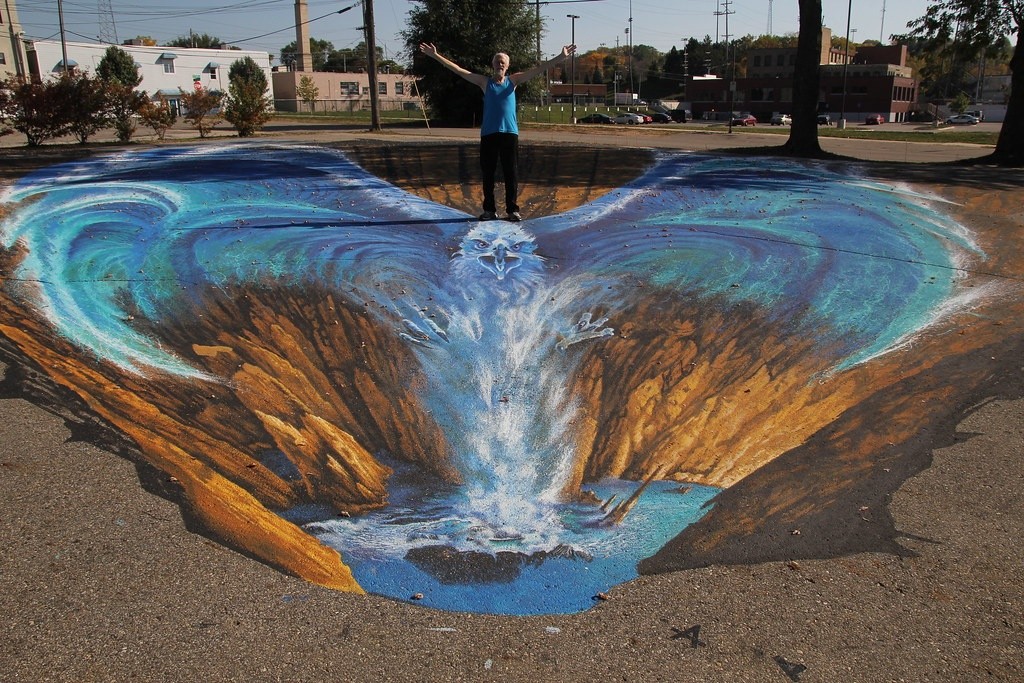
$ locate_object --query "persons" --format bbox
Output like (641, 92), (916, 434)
(420, 42), (578, 221)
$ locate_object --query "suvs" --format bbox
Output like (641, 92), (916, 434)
(959, 110), (985, 122)
(665, 109), (692, 123)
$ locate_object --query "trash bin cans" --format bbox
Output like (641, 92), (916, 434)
(974, 111), (983, 121)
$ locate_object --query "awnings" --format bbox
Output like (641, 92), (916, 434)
(208, 62), (218, 68)
(59, 60), (78, 66)
(159, 88), (181, 95)
(161, 53), (177, 59)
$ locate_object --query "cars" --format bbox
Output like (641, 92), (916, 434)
(577, 113), (615, 124)
(943, 114), (979, 124)
(817, 115), (834, 126)
(613, 113), (672, 124)
(770, 114), (792, 125)
(865, 114), (884, 125)
(732, 114), (759, 126)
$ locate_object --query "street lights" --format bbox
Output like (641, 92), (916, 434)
(567, 14), (580, 124)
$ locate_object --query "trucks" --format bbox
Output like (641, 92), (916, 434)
(615, 93), (647, 105)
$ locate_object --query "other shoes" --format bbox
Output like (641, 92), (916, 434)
(507, 212), (522, 221)
(479, 210), (497, 220)
